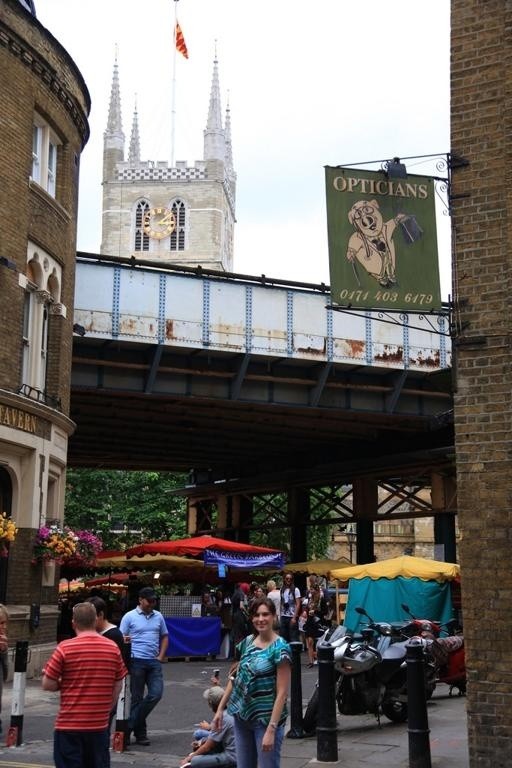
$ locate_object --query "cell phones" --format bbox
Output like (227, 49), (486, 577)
(213, 670), (220, 678)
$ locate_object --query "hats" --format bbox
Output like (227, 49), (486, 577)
(202, 685), (226, 704)
(137, 585), (160, 601)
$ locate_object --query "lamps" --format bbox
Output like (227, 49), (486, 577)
(73, 319), (86, 336)
(1, 256), (17, 270)
(383, 156), (411, 181)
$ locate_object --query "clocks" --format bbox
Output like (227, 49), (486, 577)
(141, 205), (177, 241)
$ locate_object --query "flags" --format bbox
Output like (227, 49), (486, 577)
(173, 20), (189, 60)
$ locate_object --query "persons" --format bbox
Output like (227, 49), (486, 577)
(120, 586), (170, 745)
(212, 594), (294, 768)
(202, 573), (333, 669)
(179, 686), (236, 768)
(346, 198), (406, 288)
(85, 596), (124, 747)
(0, 603), (10, 734)
(192, 661), (238, 740)
(41, 601), (123, 768)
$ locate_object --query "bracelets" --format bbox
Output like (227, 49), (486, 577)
(269, 721), (278, 729)
(188, 752), (194, 757)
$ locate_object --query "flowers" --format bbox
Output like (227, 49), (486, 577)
(71, 529), (104, 572)
(1, 513), (20, 558)
(29, 522), (80, 567)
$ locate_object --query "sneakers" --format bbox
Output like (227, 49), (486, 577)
(123, 726), (133, 747)
(135, 736), (152, 746)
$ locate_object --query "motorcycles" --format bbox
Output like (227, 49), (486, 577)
(302, 604), (466, 735)
